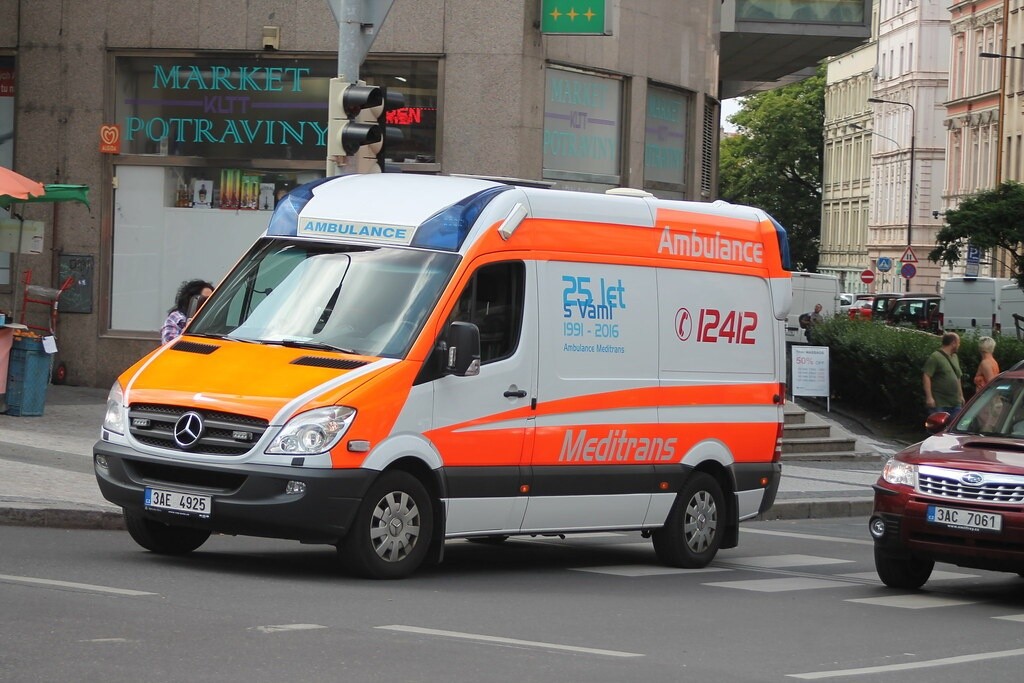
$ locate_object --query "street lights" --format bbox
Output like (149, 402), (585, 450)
(326, 78), (383, 180)
(867, 97), (915, 293)
(845, 123), (906, 249)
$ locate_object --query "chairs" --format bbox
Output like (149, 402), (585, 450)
(914, 307), (921, 317)
(900, 306), (906, 315)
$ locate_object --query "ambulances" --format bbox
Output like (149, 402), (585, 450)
(93, 163), (791, 579)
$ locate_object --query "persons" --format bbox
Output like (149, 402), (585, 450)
(921, 332), (966, 426)
(155, 276), (216, 347)
(799, 304), (823, 344)
(974, 336), (1001, 394)
(992, 321), (1003, 334)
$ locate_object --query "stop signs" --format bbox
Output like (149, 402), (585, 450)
(861, 270), (875, 283)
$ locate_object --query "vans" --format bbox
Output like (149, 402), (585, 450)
(938, 277), (1024, 339)
(786, 271), (840, 344)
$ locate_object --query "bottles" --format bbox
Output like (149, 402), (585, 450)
(176, 184), (188, 207)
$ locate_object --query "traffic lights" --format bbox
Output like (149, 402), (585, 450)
(380, 85), (404, 174)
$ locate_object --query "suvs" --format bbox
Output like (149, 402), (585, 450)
(839, 291), (941, 334)
(868, 360), (1024, 592)
